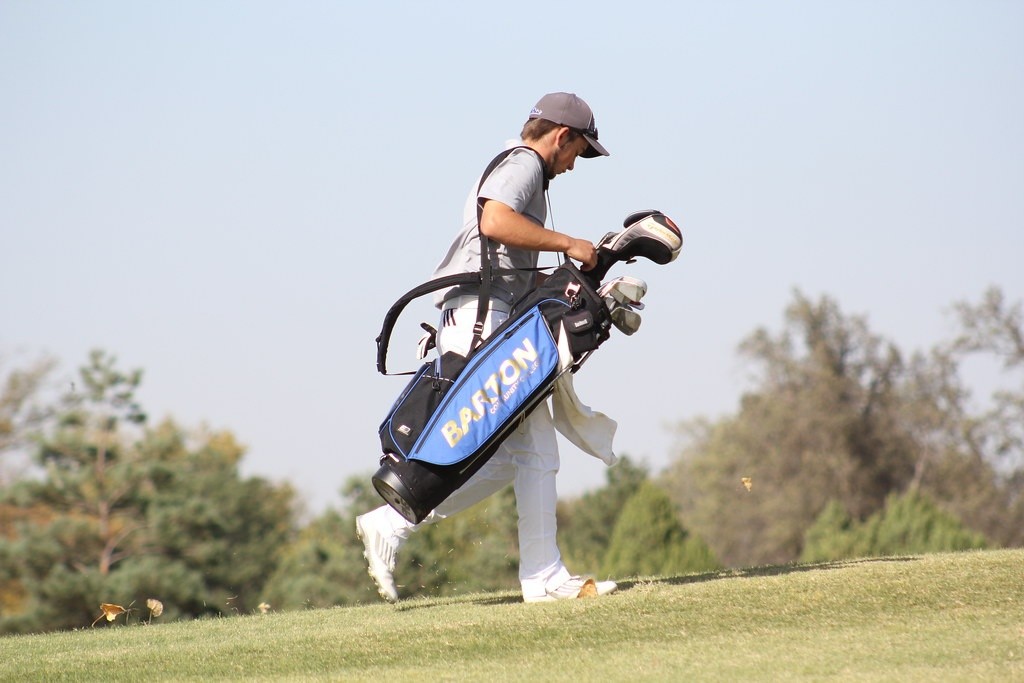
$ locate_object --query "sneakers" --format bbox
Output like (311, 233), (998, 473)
(524, 576), (617, 603)
(355, 515), (398, 604)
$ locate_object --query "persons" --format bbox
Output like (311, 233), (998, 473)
(356, 92), (622, 604)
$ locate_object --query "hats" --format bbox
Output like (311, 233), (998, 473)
(528, 91), (611, 156)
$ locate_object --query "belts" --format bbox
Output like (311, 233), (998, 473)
(441, 296), (516, 315)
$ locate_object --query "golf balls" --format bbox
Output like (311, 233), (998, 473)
(595, 275), (648, 335)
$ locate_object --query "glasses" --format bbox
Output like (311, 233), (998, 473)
(561, 124), (598, 139)
